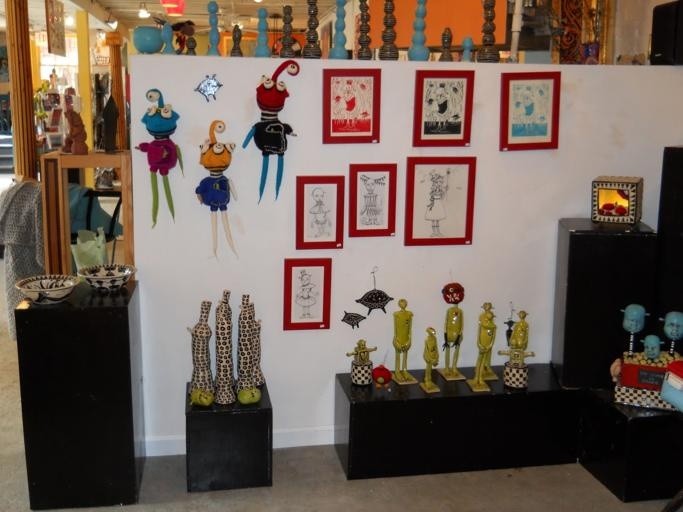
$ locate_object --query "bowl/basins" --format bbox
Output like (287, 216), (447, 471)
(77, 265), (137, 291)
(15, 274), (79, 304)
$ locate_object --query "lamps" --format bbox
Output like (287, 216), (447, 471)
(160, 0), (184, 16)
(138, 3), (151, 18)
(105, 13), (118, 29)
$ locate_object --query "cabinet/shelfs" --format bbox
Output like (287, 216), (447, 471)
(405, 155), (476, 245)
(296, 175), (345, 250)
(500, 71), (562, 151)
(413, 70), (475, 147)
(336, 360), (580, 479)
(323, 68), (380, 144)
(284, 258), (332, 331)
(349, 164), (396, 237)
(548, 221), (682, 502)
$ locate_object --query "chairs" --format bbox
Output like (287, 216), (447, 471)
(71, 191), (122, 265)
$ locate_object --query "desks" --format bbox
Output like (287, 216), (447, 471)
(186, 379), (273, 493)
(13, 281), (146, 510)
